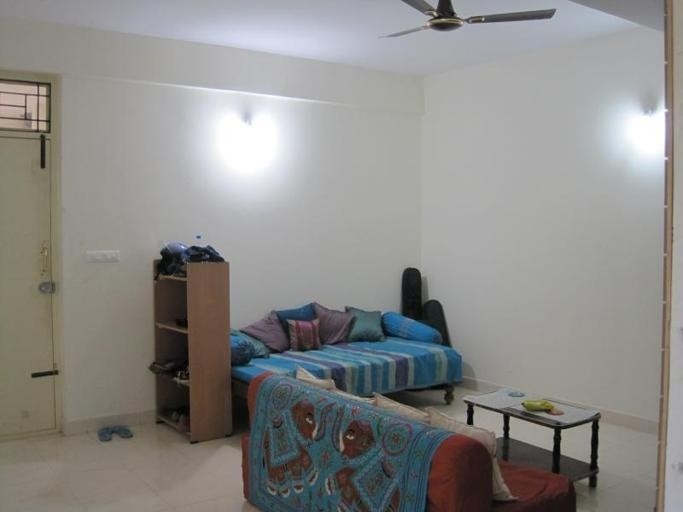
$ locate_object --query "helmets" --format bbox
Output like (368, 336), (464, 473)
(160, 242), (188, 260)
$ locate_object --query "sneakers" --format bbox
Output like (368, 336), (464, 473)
(170, 407), (189, 424)
(172, 368), (189, 384)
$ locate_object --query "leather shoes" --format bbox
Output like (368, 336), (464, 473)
(148, 358), (189, 376)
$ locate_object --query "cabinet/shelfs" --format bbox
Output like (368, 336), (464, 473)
(153, 257), (232, 445)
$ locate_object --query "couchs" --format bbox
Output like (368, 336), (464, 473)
(242, 374), (576, 512)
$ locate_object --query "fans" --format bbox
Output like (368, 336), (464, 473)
(378, 0), (556, 40)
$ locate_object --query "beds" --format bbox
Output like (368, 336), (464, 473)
(232, 335), (462, 405)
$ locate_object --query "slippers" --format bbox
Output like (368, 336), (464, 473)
(112, 425), (133, 438)
(97, 426), (112, 442)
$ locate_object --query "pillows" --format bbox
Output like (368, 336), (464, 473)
(295, 363), (519, 502)
(230, 267), (444, 364)
